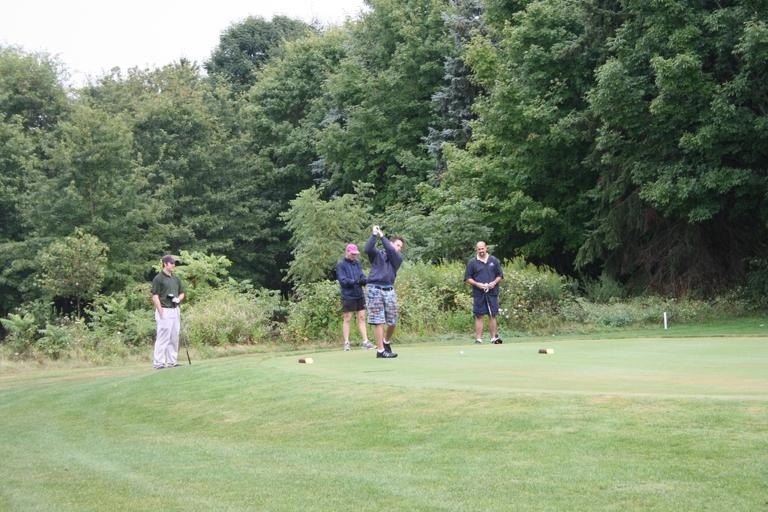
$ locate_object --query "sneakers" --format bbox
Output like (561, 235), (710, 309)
(377, 350), (398, 358)
(154, 363), (183, 369)
(383, 338), (392, 353)
(476, 338), (498, 344)
(361, 341), (377, 349)
(344, 342), (350, 351)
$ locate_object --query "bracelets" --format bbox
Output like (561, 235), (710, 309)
(493, 281), (497, 286)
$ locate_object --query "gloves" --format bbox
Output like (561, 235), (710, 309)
(359, 279), (367, 286)
(172, 297), (180, 304)
(373, 226), (380, 236)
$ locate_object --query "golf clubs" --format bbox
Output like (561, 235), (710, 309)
(483, 289), (504, 345)
(167, 292), (192, 365)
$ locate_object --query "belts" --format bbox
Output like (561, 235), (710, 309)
(367, 284), (393, 291)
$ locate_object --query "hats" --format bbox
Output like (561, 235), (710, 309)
(345, 244), (360, 255)
(162, 255), (177, 264)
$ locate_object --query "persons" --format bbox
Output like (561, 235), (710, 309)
(333, 242), (377, 352)
(461, 239), (505, 345)
(362, 223), (406, 360)
(148, 253), (189, 369)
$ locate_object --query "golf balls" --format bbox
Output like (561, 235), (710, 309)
(459, 350), (465, 355)
